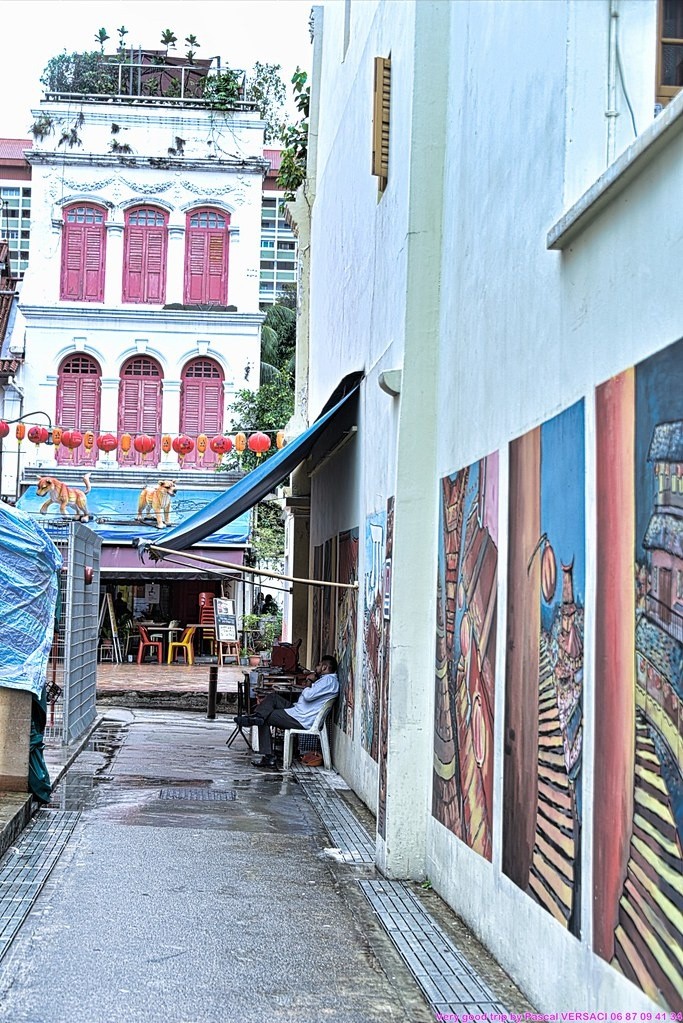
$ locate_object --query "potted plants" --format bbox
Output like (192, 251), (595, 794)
(238, 84), (244, 94)
(275, 624), (282, 641)
(239, 647), (249, 665)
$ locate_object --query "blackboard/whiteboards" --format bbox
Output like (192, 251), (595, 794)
(213, 598), (239, 642)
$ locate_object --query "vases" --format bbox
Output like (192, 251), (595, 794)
(249, 655), (261, 667)
(262, 659), (271, 667)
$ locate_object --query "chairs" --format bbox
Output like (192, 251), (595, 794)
(199, 592), (215, 655)
(136, 620), (162, 665)
(225, 670), (335, 772)
(167, 626), (196, 665)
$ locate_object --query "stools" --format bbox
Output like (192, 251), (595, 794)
(100, 644), (124, 662)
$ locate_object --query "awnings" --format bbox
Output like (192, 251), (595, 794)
(135, 385), (361, 564)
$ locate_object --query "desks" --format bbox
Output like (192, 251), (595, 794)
(134, 622), (185, 664)
(238, 630), (261, 653)
(253, 682), (306, 702)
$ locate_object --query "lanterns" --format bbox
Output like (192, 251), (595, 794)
(1, 417), (285, 459)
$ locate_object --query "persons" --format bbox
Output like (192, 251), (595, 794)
(113, 591), (132, 619)
(262, 596), (278, 616)
(252, 592), (265, 615)
(234, 654), (341, 768)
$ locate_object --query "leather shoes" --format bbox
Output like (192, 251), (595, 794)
(251, 756), (276, 769)
(233, 715), (264, 728)
